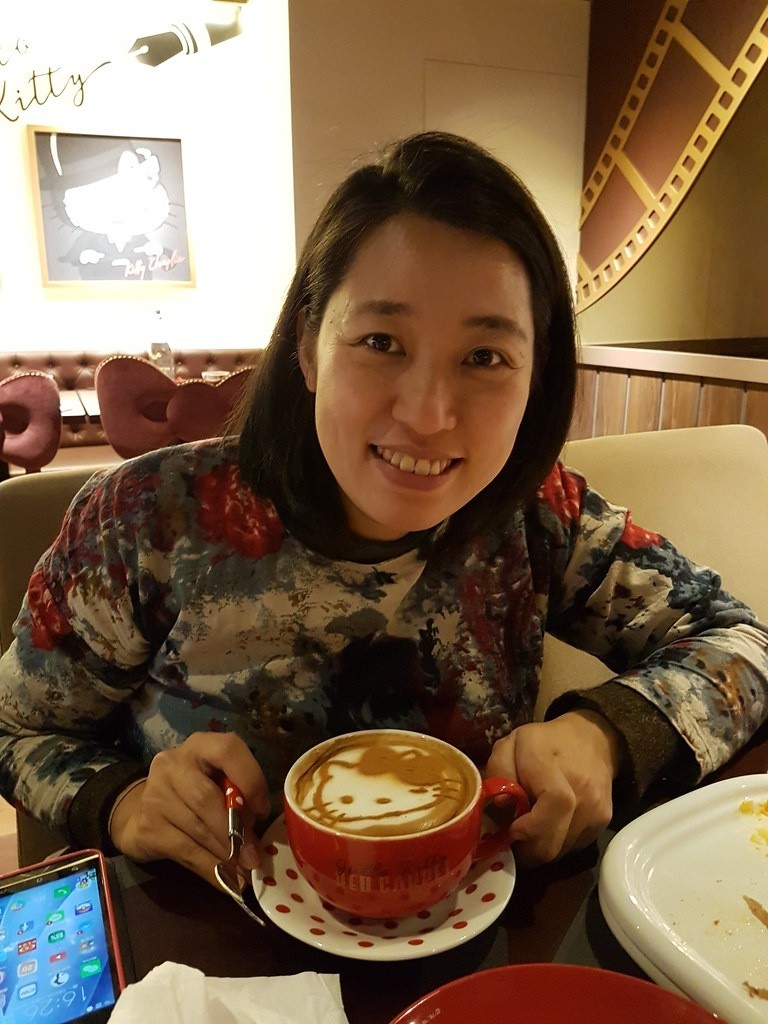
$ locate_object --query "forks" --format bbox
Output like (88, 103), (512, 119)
(213, 774), (266, 927)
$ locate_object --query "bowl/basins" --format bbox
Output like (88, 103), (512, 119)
(386, 964), (725, 1024)
(201, 371), (229, 382)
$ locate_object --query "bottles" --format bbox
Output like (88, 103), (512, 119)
(147, 309), (175, 383)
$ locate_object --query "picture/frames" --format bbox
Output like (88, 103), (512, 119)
(23, 123), (198, 292)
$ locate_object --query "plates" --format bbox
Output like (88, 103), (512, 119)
(250, 813), (517, 962)
(596, 771), (768, 1024)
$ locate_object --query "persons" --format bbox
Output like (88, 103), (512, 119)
(0, 129), (768, 895)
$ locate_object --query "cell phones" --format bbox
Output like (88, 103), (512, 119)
(0, 851), (137, 1024)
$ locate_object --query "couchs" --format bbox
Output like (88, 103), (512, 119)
(0, 349), (264, 449)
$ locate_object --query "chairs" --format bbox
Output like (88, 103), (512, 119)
(0, 467), (109, 661)
(0, 372), (62, 482)
(95, 356), (255, 461)
(530, 424), (768, 726)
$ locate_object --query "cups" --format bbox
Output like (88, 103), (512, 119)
(282, 728), (530, 920)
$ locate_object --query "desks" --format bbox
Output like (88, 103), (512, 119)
(56, 725), (768, 1024)
